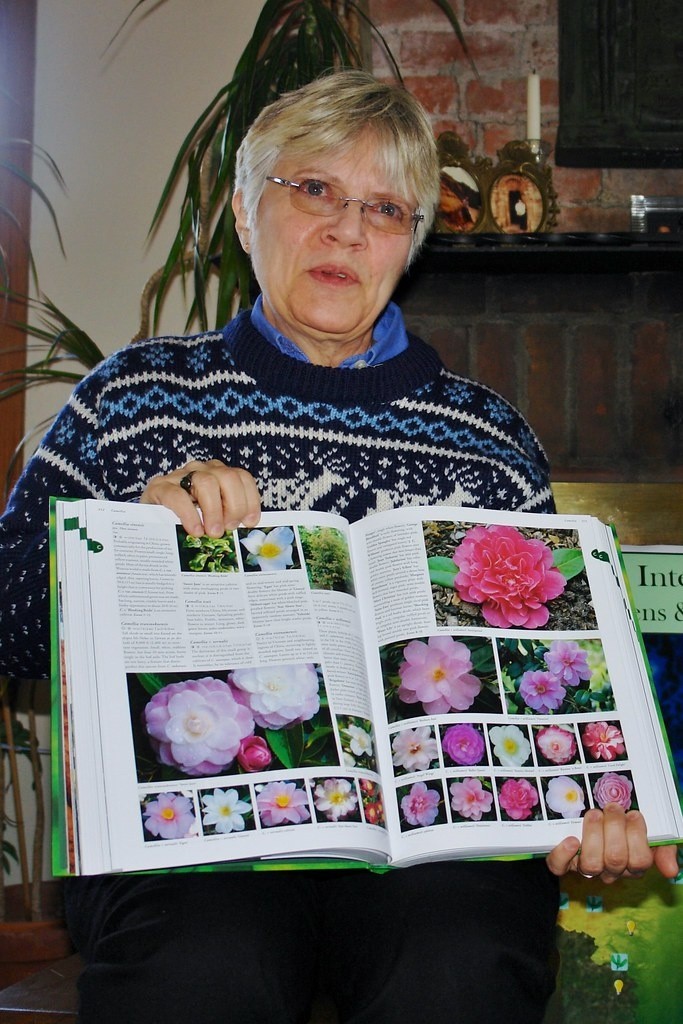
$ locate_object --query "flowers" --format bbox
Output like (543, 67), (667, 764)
(427, 525), (585, 629)
(138, 663), (386, 840)
(237, 526), (302, 572)
(381, 636), (640, 826)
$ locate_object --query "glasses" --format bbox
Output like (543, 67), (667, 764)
(264, 175), (424, 240)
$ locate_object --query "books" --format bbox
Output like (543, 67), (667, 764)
(49, 497), (683, 878)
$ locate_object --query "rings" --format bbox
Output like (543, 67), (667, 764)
(180, 471), (198, 495)
(577, 863), (601, 879)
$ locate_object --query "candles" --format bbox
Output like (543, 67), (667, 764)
(527, 68), (541, 163)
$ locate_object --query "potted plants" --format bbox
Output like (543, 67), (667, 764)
(0, 0), (480, 1024)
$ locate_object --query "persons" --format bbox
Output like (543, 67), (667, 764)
(514, 198), (526, 229)
(439, 178), (475, 231)
(0, 70), (680, 1024)
